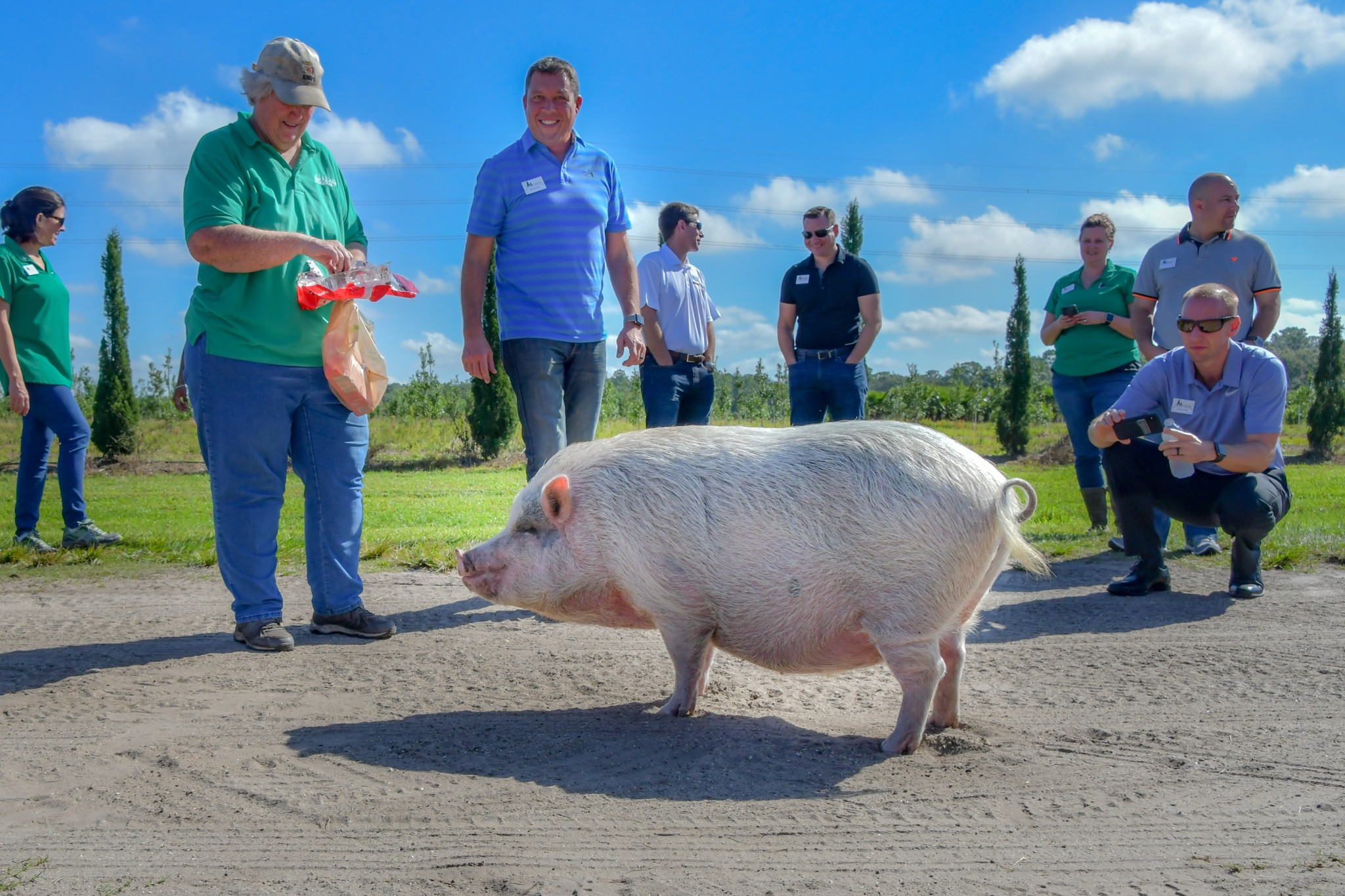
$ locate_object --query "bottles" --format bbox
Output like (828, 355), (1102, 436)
(1161, 419), (1194, 479)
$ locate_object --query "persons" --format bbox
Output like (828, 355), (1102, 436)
(173, 37), (396, 652)
(460, 58), (646, 480)
(638, 201), (721, 427)
(1040, 212), (1137, 533)
(1, 185), (124, 550)
(777, 207), (882, 426)
(1130, 172), (1282, 363)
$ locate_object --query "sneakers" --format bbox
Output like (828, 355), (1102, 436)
(13, 530), (58, 553)
(1106, 536), (1124, 549)
(310, 605), (398, 639)
(61, 519), (124, 549)
(234, 619), (295, 652)
(1186, 535), (1222, 556)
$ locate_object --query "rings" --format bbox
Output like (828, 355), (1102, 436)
(1084, 319), (1087, 323)
(640, 356), (643, 358)
(1177, 447), (1180, 455)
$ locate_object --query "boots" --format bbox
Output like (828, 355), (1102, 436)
(1079, 488), (1109, 536)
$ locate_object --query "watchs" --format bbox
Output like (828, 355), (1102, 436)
(1208, 442), (1226, 463)
(703, 362), (715, 369)
(623, 313), (645, 327)
(1088, 283), (1291, 601)
(1104, 312), (1114, 326)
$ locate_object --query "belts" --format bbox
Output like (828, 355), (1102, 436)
(797, 343), (853, 359)
(669, 350), (706, 363)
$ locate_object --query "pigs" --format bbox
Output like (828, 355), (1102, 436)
(461, 415), (1050, 755)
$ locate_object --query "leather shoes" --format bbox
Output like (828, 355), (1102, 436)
(1108, 561), (1172, 596)
(1228, 558), (1265, 597)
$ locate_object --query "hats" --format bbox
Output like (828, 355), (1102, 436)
(253, 37), (332, 111)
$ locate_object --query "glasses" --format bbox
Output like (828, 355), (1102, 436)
(687, 220), (702, 230)
(1176, 314), (1237, 333)
(802, 226), (834, 238)
(48, 215), (66, 225)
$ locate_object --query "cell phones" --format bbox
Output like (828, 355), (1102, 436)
(1111, 414), (1163, 440)
(1061, 304), (1079, 316)
